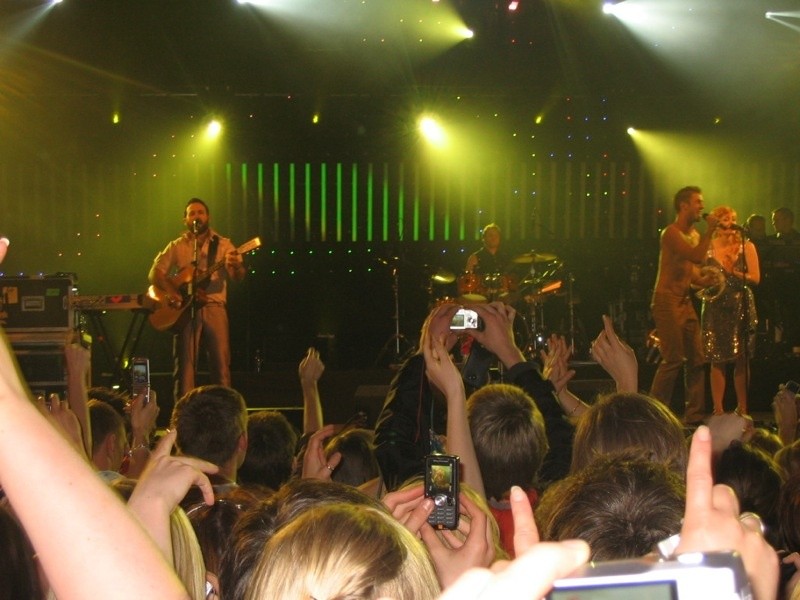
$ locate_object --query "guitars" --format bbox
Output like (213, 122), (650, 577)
(144, 237), (262, 333)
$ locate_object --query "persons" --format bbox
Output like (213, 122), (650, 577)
(650, 185), (720, 426)
(690, 205), (760, 418)
(149, 198), (244, 403)
(466, 223), (513, 275)
(0, 237), (800, 600)
(746, 208), (800, 354)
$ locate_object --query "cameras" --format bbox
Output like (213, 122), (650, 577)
(446, 309), (479, 330)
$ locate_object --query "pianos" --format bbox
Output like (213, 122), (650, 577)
(71, 290), (156, 389)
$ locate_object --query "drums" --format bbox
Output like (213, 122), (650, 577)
(459, 272), (491, 303)
(487, 271), (513, 297)
(513, 309), (532, 356)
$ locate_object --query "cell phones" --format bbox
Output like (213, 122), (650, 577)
(424, 453), (460, 531)
(132, 358), (150, 403)
(543, 548), (757, 600)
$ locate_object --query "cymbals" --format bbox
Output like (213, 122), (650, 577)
(375, 254), (416, 273)
(431, 263), (459, 285)
(694, 264), (726, 303)
(523, 278), (562, 304)
(511, 249), (557, 267)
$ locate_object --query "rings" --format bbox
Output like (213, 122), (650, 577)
(740, 513), (765, 534)
(325, 463), (334, 472)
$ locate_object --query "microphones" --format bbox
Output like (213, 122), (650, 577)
(192, 219), (200, 233)
(729, 224), (760, 240)
(702, 213), (726, 229)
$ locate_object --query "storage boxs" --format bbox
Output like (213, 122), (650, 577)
(0, 272), (78, 344)
